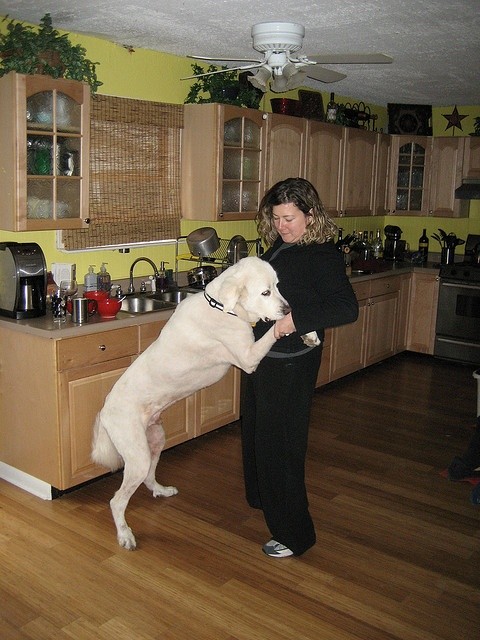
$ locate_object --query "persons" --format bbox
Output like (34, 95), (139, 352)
(239, 178), (358, 557)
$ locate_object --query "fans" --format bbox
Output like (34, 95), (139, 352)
(175, 21), (394, 91)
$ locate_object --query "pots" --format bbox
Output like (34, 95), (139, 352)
(185, 227), (221, 258)
(187, 265), (218, 289)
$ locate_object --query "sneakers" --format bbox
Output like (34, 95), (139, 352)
(262, 540), (294, 558)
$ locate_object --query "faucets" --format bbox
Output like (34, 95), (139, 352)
(128, 257), (159, 292)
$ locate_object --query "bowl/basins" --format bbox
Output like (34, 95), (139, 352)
(67, 299), (98, 317)
(95, 299), (122, 319)
(83, 291), (111, 311)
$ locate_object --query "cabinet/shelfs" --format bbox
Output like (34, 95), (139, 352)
(404, 265), (439, 356)
(264, 115), (344, 222)
(464, 133), (480, 183)
(1, 72), (93, 233)
(345, 124), (389, 217)
(4, 308), (240, 490)
(390, 134), (432, 217)
(431, 136), (470, 218)
(316, 267), (409, 388)
(180, 102), (262, 223)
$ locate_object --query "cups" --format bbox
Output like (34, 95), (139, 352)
(441, 247), (455, 266)
(50, 288), (68, 323)
(71, 297), (98, 324)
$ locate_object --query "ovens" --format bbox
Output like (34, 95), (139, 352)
(433, 283), (480, 367)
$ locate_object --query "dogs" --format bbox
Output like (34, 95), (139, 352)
(88, 255), (301, 552)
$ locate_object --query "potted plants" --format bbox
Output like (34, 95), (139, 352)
(1, 13), (104, 96)
(183, 61), (264, 111)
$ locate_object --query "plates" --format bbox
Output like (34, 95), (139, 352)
(226, 235), (249, 263)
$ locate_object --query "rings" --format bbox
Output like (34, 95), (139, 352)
(285, 334), (291, 337)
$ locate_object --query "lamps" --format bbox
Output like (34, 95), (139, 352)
(245, 49), (313, 94)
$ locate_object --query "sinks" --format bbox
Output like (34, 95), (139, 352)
(120, 295), (176, 317)
(153, 289), (199, 304)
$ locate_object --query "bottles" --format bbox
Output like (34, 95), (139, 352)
(368, 230), (376, 258)
(418, 228), (429, 257)
(357, 231), (365, 262)
(363, 230), (372, 260)
(325, 92), (338, 123)
(335, 227), (347, 255)
(374, 228), (383, 254)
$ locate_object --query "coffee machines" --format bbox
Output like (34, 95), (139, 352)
(0, 241), (48, 320)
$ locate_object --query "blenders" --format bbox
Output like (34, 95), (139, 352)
(383, 224), (407, 262)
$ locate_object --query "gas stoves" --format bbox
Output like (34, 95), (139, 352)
(438, 261), (479, 282)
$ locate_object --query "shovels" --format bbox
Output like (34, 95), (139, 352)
(449, 232), (457, 248)
(454, 238), (465, 248)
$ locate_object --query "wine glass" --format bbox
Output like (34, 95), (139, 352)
(60, 280), (78, 316)
